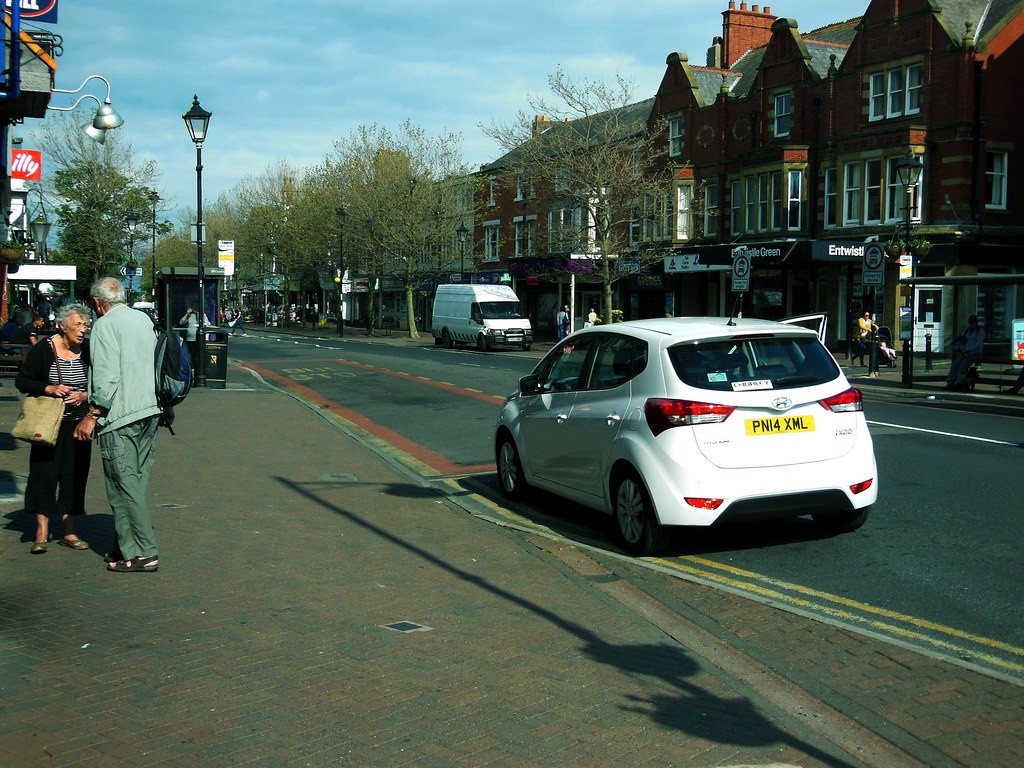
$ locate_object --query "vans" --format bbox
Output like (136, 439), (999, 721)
(431, 284), (535, 352)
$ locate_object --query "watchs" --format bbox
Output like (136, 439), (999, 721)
(87, 410), (100, 419)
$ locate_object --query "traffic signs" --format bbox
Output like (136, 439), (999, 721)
(120, 267), (143, 277)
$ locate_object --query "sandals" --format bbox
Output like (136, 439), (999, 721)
(107, 554), (159, 572)
(104, 551), (126, 563)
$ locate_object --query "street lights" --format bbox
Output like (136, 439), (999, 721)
(336, 208), (349, 333)
(895, 149), (924, 388)
(146, 189), (163, 302)
(181, 93), (212, 390)
(455, 222), (469, 283)
(126, 212), (140, 307)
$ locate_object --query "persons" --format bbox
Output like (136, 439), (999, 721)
(73, 278), (163, 572)
(0, 312), (44, 372)
(179, 302), (211, 379)
(661, 307), (672, 318)
(21, 295), (70, 330)
(945, 315), (985, 386)
(15, 303), (91, 553)
(220, 301), (308, 336)
(556, 305), (571, 342)
(850, 312), (896, 367)
(588, 308), (598, 323)
(1002, 367), (1024, 395)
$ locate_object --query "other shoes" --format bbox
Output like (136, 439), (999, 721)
(860, 365), (867, 368)
(31, 540), (48, 552)
(851, 358), (854, 364)
(59, 537), (89, 550)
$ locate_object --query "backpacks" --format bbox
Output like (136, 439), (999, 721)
(133, 308), (192, 406)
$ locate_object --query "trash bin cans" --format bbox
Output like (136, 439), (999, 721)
(194, 327), (228, 389)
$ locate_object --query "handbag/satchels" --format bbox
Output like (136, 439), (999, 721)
(10, 397), (64, 445)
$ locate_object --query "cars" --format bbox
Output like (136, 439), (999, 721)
(494, 316), (878, 557)
